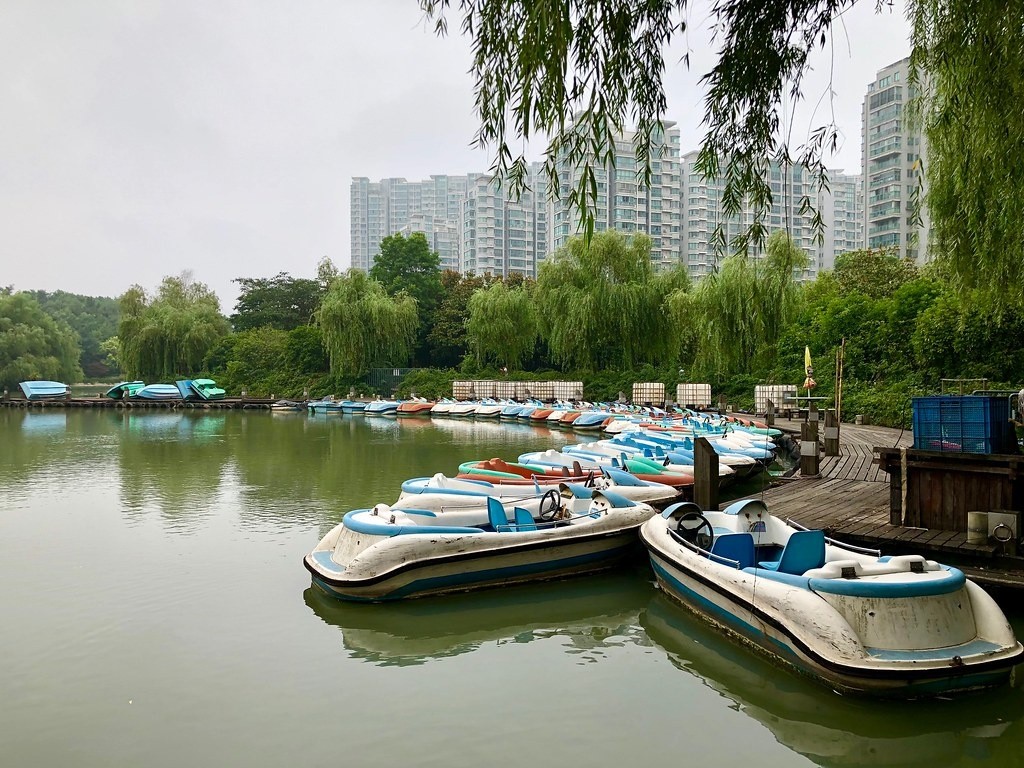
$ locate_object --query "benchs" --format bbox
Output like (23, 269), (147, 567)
(784, 406), (836, 423)
(801, 554), (941, 579)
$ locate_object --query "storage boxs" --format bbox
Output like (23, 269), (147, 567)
(911, 395), (1009, 455)
(988, 509), (1020, 540)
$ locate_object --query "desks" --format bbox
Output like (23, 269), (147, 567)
(787, 395), (832, 423)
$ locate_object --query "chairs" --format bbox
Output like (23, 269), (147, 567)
(368, 503), (419, 528)
(487, 497), (538, 533)
(708, 529), (826, 575)
(396, 398), (763, 495)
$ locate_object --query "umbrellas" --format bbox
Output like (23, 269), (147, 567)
(802, 345), (817, 420)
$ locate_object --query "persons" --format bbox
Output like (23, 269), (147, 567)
(500, 366), (508, 376)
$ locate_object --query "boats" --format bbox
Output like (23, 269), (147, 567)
(639, 591), (1013, 768)
(191, 377), (227, 400)
(136, 383), (183, 400)
(175, 380), (201, 401)
(301, 566), (653, 668)
(637, 497), (1024, 707)
(300, 506), (654, 602)
(18, 380), (71, 402)
(270, 401), (301, 411)
(306, 400), (785, 504)
(106, 381), (146, 400)
